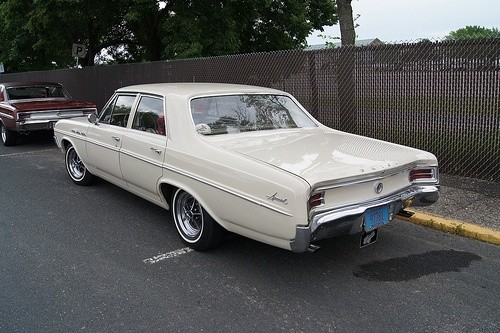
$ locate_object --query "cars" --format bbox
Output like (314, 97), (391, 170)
(54, 82), (440, 254)
(0, 81), (97, 148)
